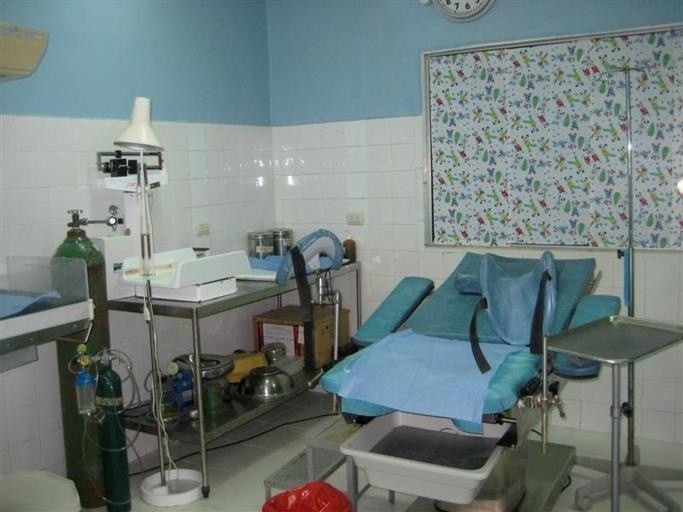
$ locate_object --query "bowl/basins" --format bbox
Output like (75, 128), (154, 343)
(235, 365), (294, 402)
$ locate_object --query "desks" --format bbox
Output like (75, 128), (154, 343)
(540, 312), (682, 511)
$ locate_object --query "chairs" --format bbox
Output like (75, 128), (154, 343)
(318, 250), (621, 511)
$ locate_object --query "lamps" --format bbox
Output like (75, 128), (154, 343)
(110, 95), (206, 510)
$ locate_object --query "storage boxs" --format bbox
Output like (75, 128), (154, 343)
(338, 411), (513, 505)
(251, 304), (350, 370)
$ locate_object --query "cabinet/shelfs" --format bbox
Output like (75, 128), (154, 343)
(106, 260), (363, 499)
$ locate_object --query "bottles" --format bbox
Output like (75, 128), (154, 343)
(152, 372), (184, 429)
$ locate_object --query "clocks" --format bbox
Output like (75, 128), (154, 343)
(418, 0), (495, 25)
